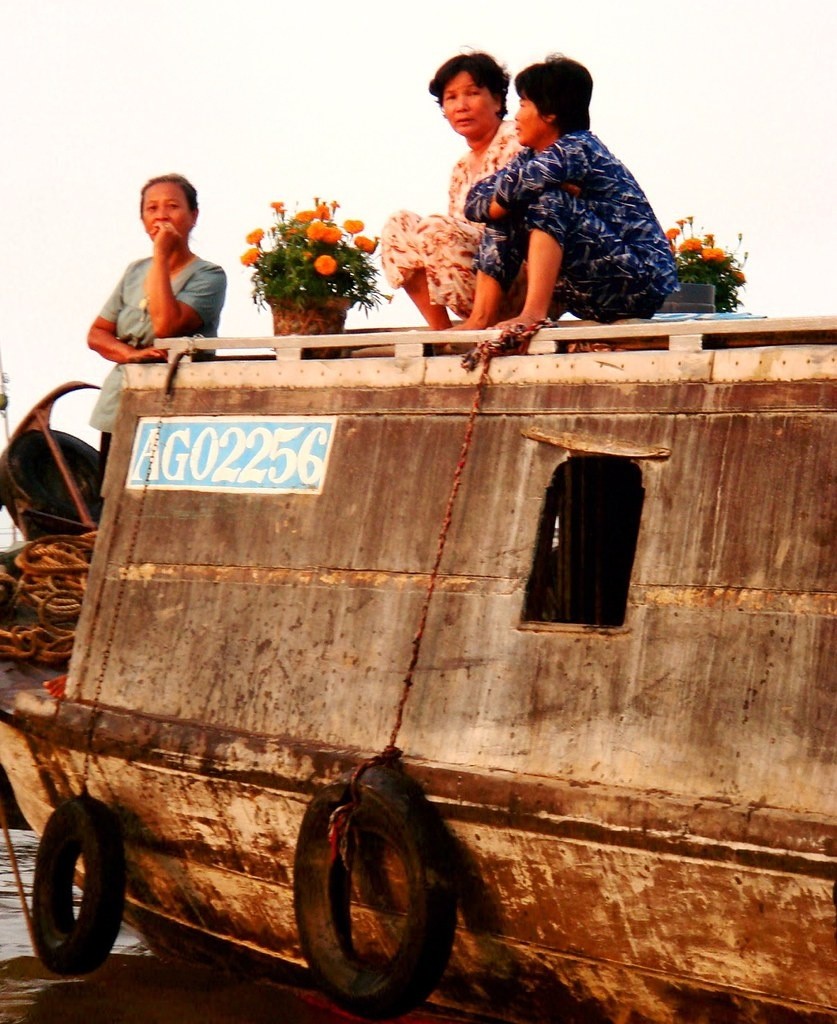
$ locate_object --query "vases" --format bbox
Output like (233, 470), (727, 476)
(652, 281), (716, 316)
(267, 296), (352, 361)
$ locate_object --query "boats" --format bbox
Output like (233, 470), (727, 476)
(0, 313), (837, 1024)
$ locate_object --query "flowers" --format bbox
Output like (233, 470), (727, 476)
(668, 215), (748, 314)
(240, 198), (394, 314)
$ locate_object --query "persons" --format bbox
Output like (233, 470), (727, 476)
(382, 51), (531, 331)
(438, 55), (677, 330)
(87, 174), (227, 503)
